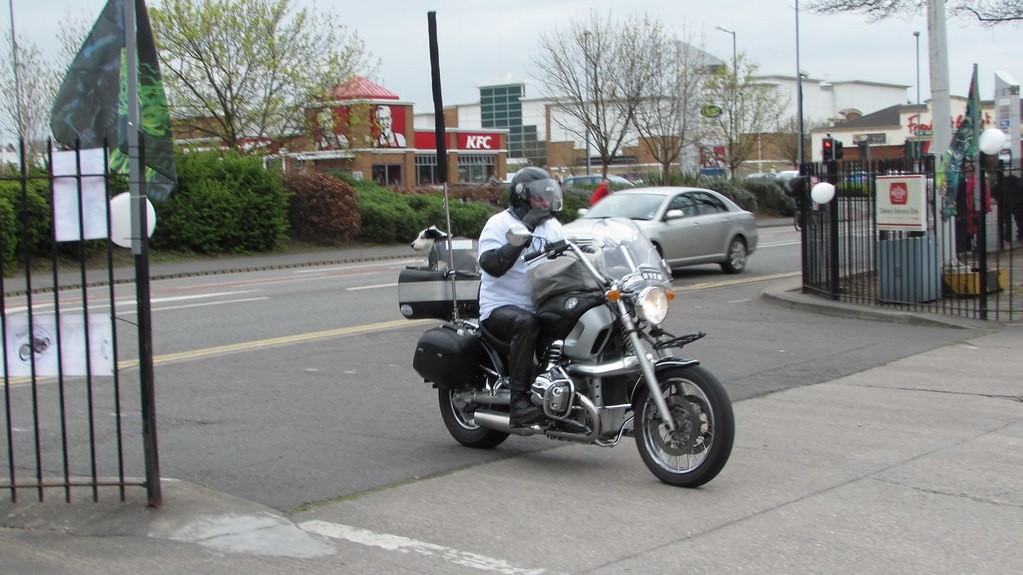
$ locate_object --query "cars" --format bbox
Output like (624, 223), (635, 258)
(563, 187), (760, 275)
(844, 171), (875, 186)
(777, 170), (800, 179)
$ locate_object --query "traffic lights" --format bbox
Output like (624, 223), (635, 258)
(834, 140), (844, 160)
(822, 137), (835, 167)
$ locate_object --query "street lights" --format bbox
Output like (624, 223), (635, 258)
(716, 25), (738, 146)
(583, 29), (591, 174)
(913, 32), (923, 173)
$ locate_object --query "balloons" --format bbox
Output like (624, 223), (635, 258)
(980, 129), (1006, 154)
(110, 192), (156, 247)
(811, 182), (836, 204)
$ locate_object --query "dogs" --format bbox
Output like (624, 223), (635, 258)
(410, 224), (479, 274)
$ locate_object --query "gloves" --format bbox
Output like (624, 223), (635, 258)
(606, 248), (628, 267)
(522, 206), (552, 230)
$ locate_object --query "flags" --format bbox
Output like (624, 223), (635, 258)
(50, 0), (178, 202)
(934, 73), (984, 216)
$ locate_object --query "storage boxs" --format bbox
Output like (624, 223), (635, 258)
(943, 268), (1005, 296)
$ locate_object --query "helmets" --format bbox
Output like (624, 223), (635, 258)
(509, 166), (552, 208)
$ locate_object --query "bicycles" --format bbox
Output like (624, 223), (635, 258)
(793, 208), (821, 231)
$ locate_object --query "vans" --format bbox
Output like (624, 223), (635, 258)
(562, 175), (633, 187)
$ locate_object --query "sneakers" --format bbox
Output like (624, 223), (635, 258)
(508, 389), (544, 427)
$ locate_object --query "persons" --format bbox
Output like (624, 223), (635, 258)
(478, 167), (563, 428)
(589, 180), (610, 206)
(966, 166), (974, 177)
(490, 170), (502, 186)
(554, 173), (562, 186)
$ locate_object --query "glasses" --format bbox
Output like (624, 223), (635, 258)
(529, 192), (544, 202)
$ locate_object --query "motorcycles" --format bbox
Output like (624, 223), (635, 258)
(412, 216), (735, 489)
